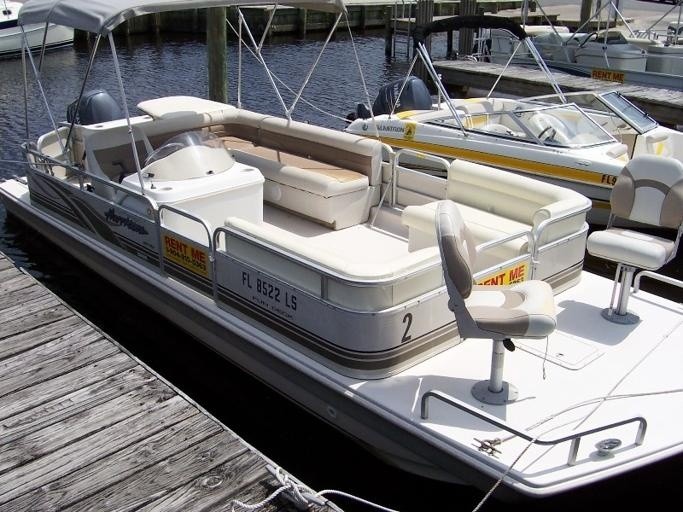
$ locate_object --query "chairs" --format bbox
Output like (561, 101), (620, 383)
(436, 200), (554, 405)
(587, 155), (683, 324)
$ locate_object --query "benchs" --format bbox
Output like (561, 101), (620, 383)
(86, 107), (383, 231)
(402, 161), (591, 297)
(488, 25), (661, 58)
(226, 218), (444, 310)
